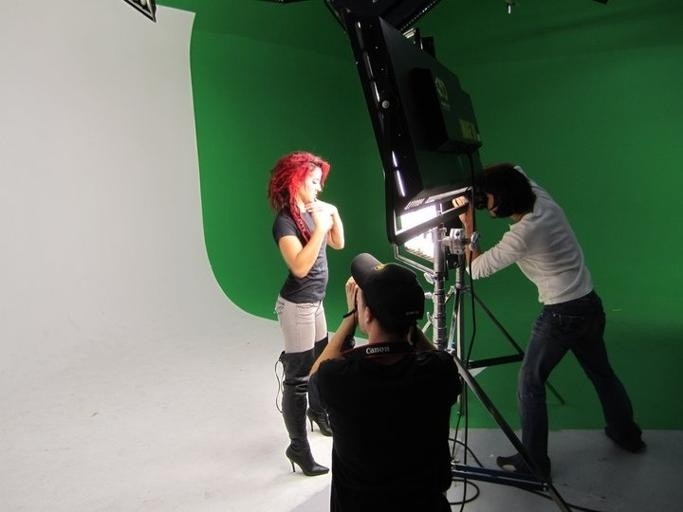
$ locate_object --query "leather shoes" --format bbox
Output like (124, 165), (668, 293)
(604, 425), (646, 453)
(496, 453), (551, 473)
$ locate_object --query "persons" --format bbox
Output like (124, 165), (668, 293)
(454, 164), (646, 476)
(308, 250), (460, 512)
(268, 152), (347, 475)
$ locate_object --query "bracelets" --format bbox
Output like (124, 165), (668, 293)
(464, 239), (472, 245)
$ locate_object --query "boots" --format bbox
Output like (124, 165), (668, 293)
(307, 333), (334, 436)
(275, 347), (329, 476)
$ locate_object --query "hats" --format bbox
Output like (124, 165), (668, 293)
(352, 253), (424, 319)
